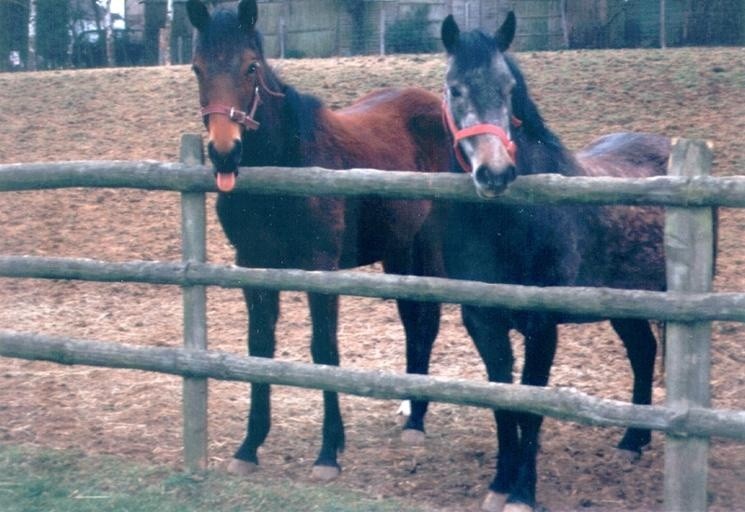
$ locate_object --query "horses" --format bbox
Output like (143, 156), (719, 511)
(441, 10), (720, 512)
(182, 0), (456, 486)
